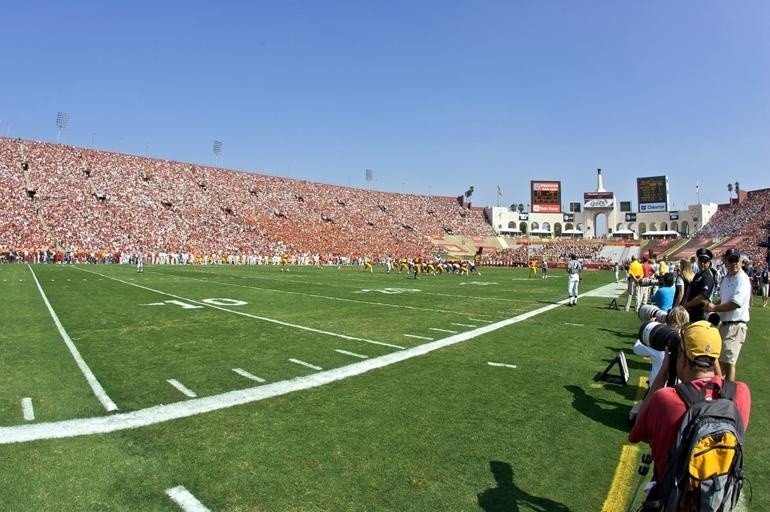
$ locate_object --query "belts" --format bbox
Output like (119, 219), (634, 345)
(723, 320), (746, 325)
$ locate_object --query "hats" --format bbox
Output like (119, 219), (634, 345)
(681, 320), (722, 367)
(696, 248), (713, 261)
(725, 248), (741, 258)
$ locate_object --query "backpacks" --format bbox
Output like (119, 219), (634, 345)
(663, 377), (744, 512)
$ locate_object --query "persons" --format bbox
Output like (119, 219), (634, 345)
(0, 137), (769, 512)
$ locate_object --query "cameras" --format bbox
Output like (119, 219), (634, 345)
(638, 304), (671, 323)
(639, 275), (659, 287)
(639, 321), (684, 351)
